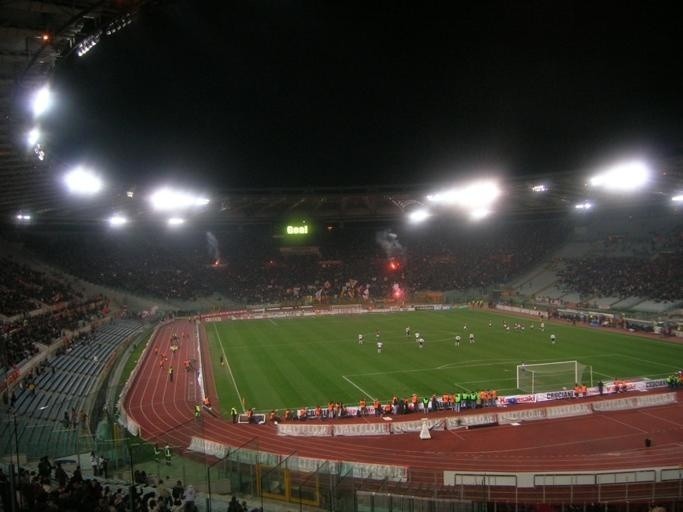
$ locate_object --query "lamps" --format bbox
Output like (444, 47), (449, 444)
(103, 12), (131, 34)
(75, 33), (100, 58)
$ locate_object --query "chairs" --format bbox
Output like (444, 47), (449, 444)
(163, 290), (232, 309)
(511, 259), (681, 314)
(0, 308), (143, 461)
(82, 472), (231, 511)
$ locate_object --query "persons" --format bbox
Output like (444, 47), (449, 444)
(2, 238), (683, 510)
